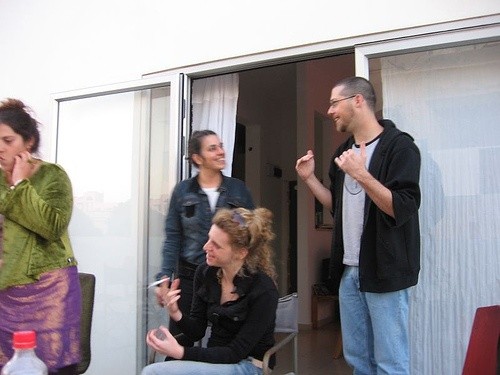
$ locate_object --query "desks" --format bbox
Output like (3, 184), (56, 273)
(310, 283), (339, 330)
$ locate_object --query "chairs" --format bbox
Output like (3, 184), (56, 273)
(148, 293), (300, 375)
(49, 272), (96, 375)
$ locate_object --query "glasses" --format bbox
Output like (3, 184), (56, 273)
(229, 212), (251, 245)
(328, 95), (355, 107)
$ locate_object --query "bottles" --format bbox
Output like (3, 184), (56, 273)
(1, 331), (48, 375)
